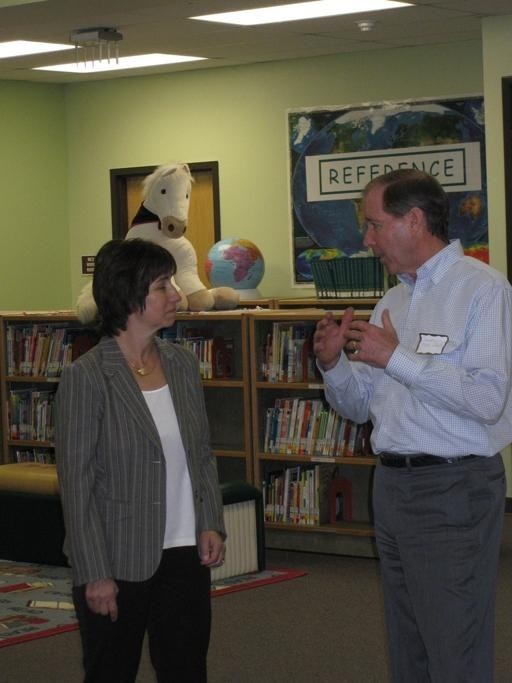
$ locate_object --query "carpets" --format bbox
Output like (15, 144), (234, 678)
(0, 558), (309, 646)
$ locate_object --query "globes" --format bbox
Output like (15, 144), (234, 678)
(205, 239), (265, 301)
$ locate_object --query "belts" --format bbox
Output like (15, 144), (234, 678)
(377, 451), (480, 467)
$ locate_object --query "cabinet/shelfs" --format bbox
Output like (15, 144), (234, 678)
(0, 297), (383, 561)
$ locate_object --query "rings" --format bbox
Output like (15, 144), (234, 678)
(350, 340), (358, 350)
(219, 554), (226, 564)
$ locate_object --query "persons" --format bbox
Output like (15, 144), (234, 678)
(54, 237), (229, 682)
(308, 167), (511, 682)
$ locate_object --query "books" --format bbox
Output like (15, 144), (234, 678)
(259, 393), (374, 529)
(158, 321), (236, 381)
(309, 254), (398, 300)
(3, 318), (99, 465)
(258, 319), (321, 383)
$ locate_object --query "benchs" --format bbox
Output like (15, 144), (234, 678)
(0, 457), (267, 585)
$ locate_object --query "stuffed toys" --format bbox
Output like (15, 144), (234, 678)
(71, 159), (241, 324)
